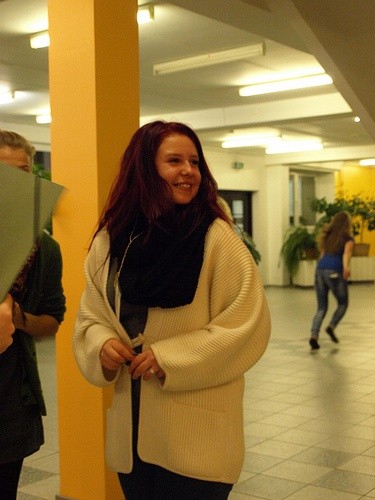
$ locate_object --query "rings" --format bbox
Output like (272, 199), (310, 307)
(149, 368), (156, 374)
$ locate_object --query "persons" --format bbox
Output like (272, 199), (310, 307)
(71, 121), (272, 500)
(309, 209), (355, 350)
(0, 130), (66, 500)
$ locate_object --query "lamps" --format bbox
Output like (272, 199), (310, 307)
(0, 90), (15, 104)
(29, 4), (156, 50)
(239, 74), (333, 97)
(153, 42), (264, 76)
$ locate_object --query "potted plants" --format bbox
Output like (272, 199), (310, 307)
(277, 195), (375, 270)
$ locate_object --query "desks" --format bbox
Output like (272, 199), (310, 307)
(291, 256), (375, 288)
